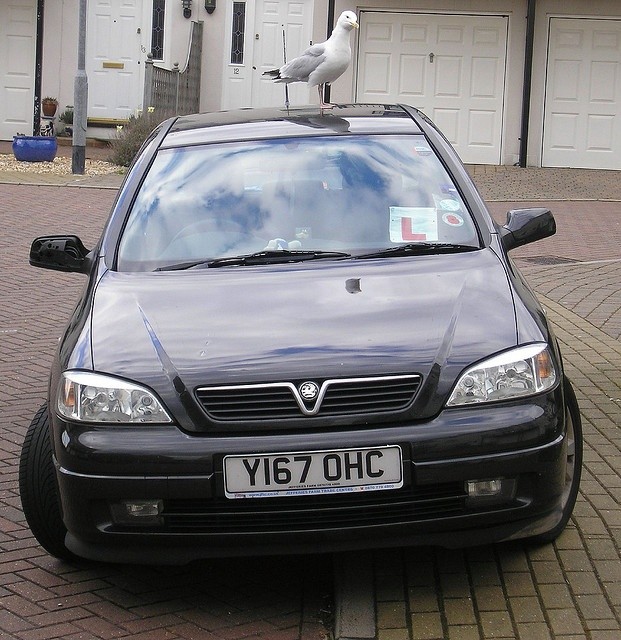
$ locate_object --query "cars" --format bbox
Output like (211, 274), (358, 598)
(19, 30), (582, 568)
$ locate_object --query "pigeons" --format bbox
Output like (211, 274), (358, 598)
(262, 11), (359, 110)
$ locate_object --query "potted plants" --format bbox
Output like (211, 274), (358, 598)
(42, 97), (58, 115)
(62, 112), (74, 136)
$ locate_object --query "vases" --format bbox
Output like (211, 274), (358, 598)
(12, 134), (57, 161)
